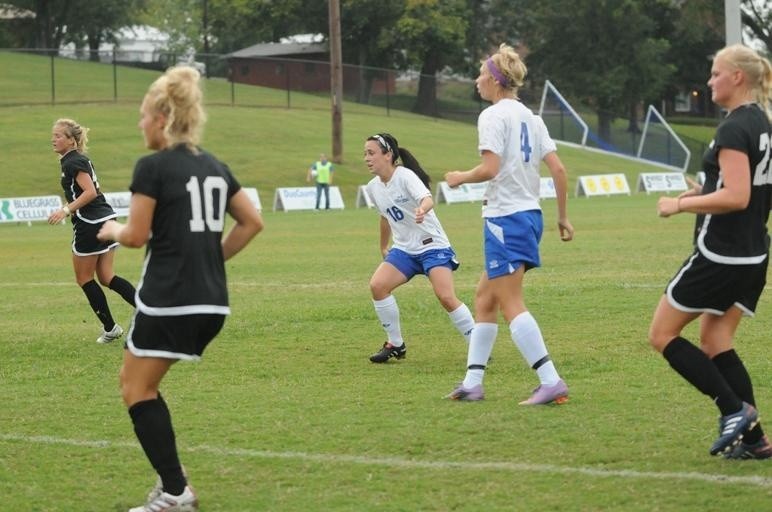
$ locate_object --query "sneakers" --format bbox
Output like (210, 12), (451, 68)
(97, 323), (124, 345)
(128, 464), (200, 512)
(711, 401), (770, 460)
(442, 383), (484, 402)
(518, 378), (569, 407)
(370, 341), (406, 363)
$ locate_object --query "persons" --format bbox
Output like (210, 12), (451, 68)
(446, 40), (572, 406)
(306, 153), (334, 212)
(365, 133), (491, 364)
(96, 63), (263, 512)
(48, 119), (137, 344)
(649, 46), (772, 459)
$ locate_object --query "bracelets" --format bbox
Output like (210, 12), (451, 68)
(62, 206), (70, 217)
(678, 198), (681, 212)
